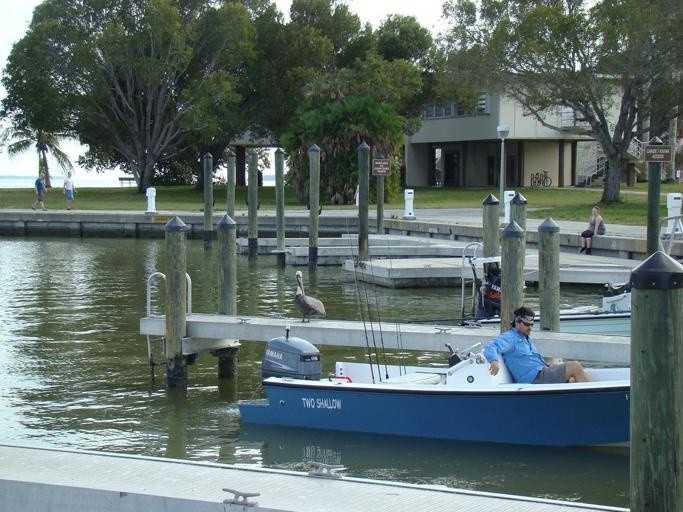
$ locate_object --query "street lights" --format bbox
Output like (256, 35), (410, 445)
(496, 124), (511, 214)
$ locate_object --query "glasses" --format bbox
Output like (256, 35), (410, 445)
(522, 320), (534, 327)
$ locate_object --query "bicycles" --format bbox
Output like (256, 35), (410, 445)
(531, 168), (552, 188)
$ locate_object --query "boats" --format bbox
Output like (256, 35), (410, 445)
(237, 327), (630, 449)
(460, 279), (632, 335)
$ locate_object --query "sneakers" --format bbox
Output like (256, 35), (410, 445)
(580, 247), (591, 254)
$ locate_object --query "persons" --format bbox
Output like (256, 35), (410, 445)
(483, 306), (593, 384)
(486, 262), (501, 317)
(32, 173), (47, 211)
(580, 206), (605, 255)
(63, 171), (78, 210)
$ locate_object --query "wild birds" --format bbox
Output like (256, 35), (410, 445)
(294, 270), (327, 323)
